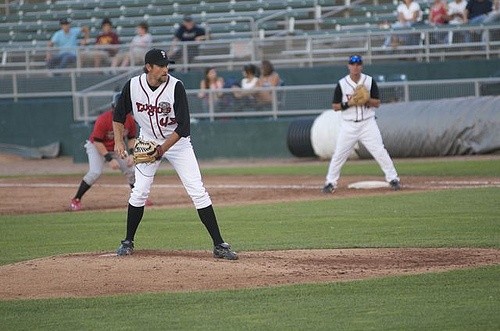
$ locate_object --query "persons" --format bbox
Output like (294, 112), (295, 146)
(411, 0), (500, 44)
(322, 54), (403, 192)
(111, 21), (152, 67)
(112, 48), (239, 260)
(166, 16), (207, 72)
(46, 17), (90, 81)
(68, 94), (153, 211)
(197, 67), (229, 114)
(231, 61), (281, 108)
(80, 18), (121, 69)
(382, 0), (424, 47)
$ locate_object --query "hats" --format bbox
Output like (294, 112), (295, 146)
(183, 14), (192, 22)
(348, 54), (362, 64)
(59, 18), (71, 24)
(144, 48), (176, 66)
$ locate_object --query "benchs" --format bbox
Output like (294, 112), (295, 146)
(0, 0), (500, 110)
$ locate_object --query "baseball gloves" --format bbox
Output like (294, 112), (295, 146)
(133, 137), (159, 164)
(355, 85), (369, 105)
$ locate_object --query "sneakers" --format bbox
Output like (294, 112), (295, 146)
(70, 198), (80, 208)
(214, 243), (238, 260)
(144, 200), (152, 206)
(117, 240), (135, 256)
(321, 182), (337, 193)
(389, 179), (400, 190)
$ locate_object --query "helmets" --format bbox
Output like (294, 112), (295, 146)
(111, 93), (121, 103)
